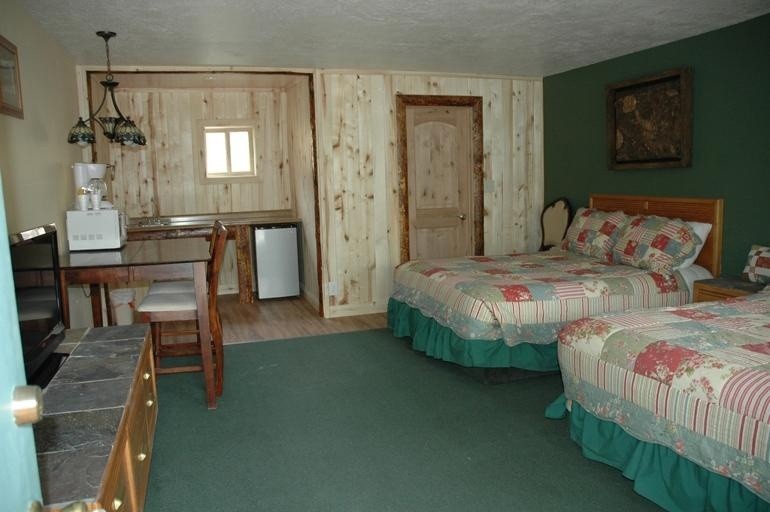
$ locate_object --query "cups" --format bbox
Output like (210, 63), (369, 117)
(78, 195), (89, 210)
(90, 194), (102, 211)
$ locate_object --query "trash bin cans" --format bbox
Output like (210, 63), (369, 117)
(108, 288), (136, 327)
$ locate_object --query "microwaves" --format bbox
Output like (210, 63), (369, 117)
(66, 206), (129, 252)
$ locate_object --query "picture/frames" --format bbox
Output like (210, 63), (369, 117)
(0, 34), (25, 121)
(604, 67), (693, 171)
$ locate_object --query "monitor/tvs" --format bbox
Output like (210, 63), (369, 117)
(9, 222), (70, 389)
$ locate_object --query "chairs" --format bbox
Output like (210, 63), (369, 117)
(136, 218), (229, 399)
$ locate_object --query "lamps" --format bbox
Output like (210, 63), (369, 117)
(63, 28), (147, 150)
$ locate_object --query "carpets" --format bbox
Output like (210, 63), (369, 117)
(144, 326), (666, 512)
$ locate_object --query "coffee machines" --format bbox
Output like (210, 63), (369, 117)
(72, 162), (115, 210)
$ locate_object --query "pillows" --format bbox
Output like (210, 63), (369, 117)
(742, 242), (770, 284)
(559, 205), (713, 272)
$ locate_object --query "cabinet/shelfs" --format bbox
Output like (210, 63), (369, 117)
(88, 321), (159, 512)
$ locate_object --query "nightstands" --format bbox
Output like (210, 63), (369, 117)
(691, 273), (765, 305)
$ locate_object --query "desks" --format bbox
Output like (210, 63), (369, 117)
(55, 234), (227, 411)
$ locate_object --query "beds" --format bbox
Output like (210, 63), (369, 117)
(394, 194), (724, 384)
(557, 284), (769, 511)
